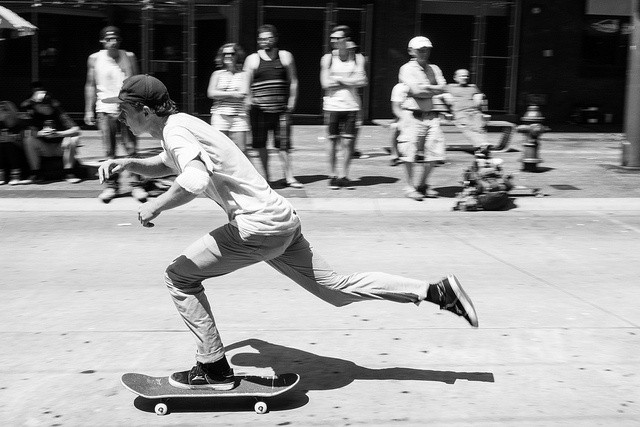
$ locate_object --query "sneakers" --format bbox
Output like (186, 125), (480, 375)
(98, 181), (119, 201)
(337, 177), (355, 189)
(405, 187), (424, 198)
(352, 152), (370, 159)
(417, 186), (439, 195)
(0, 172), (5, 184)
(437, 273), (478, 327)
(168, 361), (236, 391)
(18, 175), (43, 184)
(8, 169), (20, 185)
(328, 177), (340, 189)
(474, 149), (488, 159)
(127, 181), (149, 200)
(286, 178), (304, 188)
(61, 174), (82, 183)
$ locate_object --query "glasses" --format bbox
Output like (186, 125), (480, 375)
(329, 37), (345, 40)
(103, 38), (121, 41)
(223, 53), (235, 56)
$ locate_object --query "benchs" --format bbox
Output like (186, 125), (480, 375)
(371, 114), (519, 157)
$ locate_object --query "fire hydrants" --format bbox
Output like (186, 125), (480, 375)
(515, 104), (551, 171)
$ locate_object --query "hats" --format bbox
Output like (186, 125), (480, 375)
(28, 91), (51, 104)
(99, 26), (121, 37)
(408, 36), (433, 50)
(345, 41), (357, 48)
(102, 74), (169, 105)
(257, 24), (277, 34)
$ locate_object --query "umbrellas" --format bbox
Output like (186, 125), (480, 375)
(0, 5), (38, 40)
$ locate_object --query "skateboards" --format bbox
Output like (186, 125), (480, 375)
(121, 372), (300, 414)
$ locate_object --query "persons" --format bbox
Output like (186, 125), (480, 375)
(389, 81), (448, 165)
(434, 68), (493, 158)
(397, 35), (440, 198)
(320, 26), (369, 190)
(96, 73), (478, 390)
(84, 24), (150, 202)
(18, 91), (80, 187)
(330, 42), (369, 160)
(207, 43), (247, 159)
(241, 23), (305, 190)
(1, 99), (30, 185)
